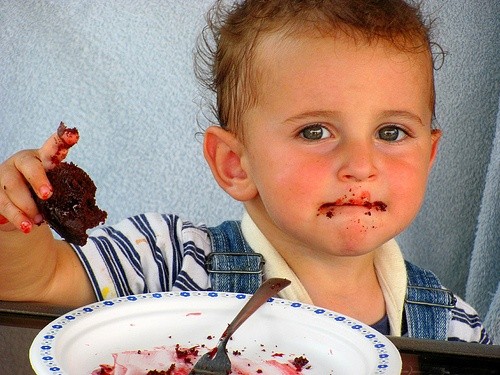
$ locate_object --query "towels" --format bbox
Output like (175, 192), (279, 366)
(0, 0), (500, 345)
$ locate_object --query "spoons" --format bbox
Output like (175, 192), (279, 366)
(188, 277), (291, 375)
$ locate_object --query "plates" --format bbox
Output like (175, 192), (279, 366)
(29, 291), (403, 375)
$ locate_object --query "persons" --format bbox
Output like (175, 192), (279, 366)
(1, 0), (496, 346)
